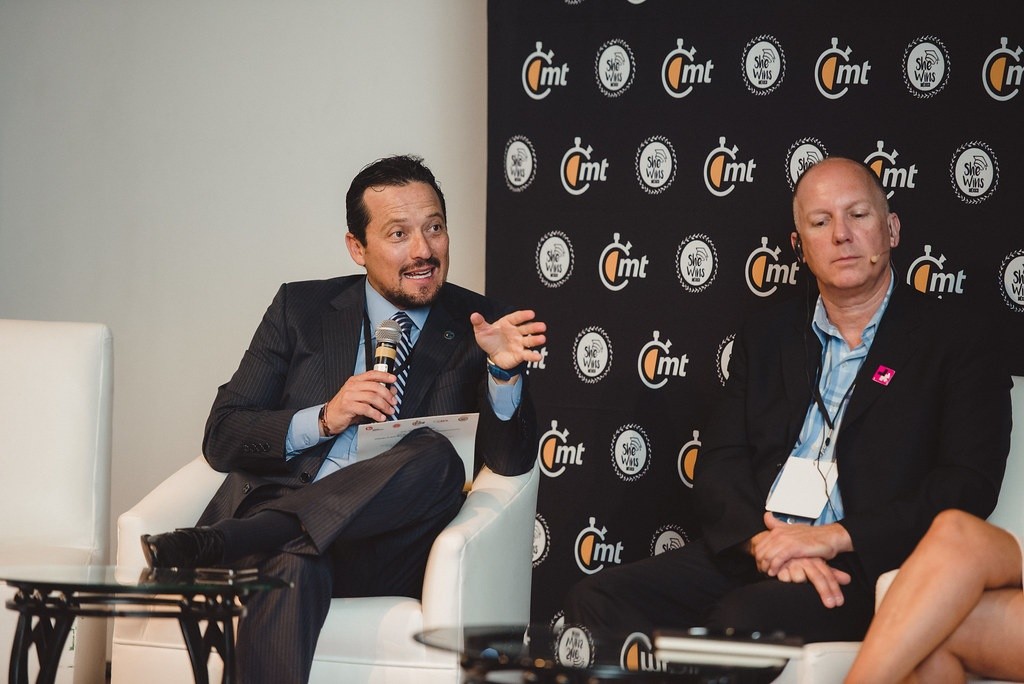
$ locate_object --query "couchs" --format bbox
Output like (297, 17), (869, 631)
(110, 449), (540, 681)
(761, 371), (1021, 683)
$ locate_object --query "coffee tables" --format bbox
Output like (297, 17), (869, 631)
(412, 624), (800, 683)
(1, 567), (286, 683)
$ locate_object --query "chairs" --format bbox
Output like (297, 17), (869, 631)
(3, 317), (114, 683)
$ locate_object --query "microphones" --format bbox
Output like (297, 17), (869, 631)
(871, 246), (890, 263)
(369, 322), (402, 411)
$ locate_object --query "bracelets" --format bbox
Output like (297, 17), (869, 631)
(319, 401), (335, 437)
(486, 357), (528, 381)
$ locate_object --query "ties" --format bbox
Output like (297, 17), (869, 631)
(377, 312), (413, 421)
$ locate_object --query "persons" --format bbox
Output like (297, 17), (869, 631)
(564, 156), (1014, 635)
(140, 155), (549, 683)
(841, 509), (1024, 683)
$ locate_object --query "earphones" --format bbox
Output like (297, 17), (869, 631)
(794, 235), (804, 261)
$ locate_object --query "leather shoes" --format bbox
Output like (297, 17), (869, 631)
(142, 526), (226, 583)
